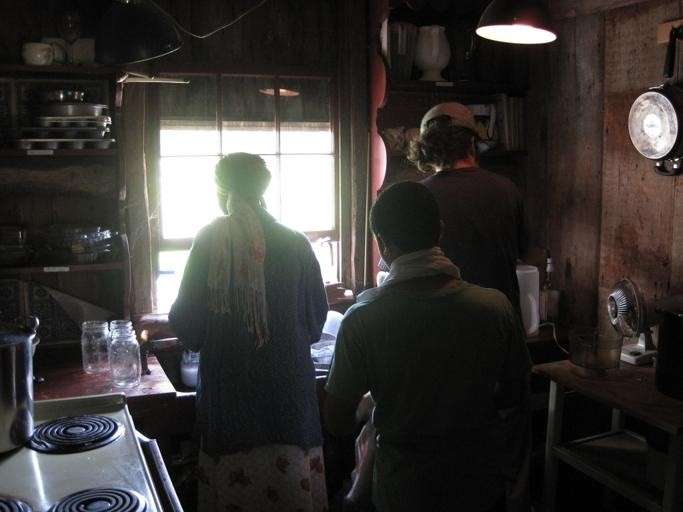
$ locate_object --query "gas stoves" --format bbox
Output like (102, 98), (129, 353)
(0, 392), (186, 512)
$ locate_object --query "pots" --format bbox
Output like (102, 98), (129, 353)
(627, 24), (683, 176)
(0, 316), (43, 453)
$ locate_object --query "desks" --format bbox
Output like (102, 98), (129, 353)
(530, 357), (682, 509)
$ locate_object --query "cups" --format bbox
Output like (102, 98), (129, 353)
(180, 350), (200, 387)
(22, 42), (54, 66)
(81, 320), (110, 375)
(108, 320), (143, 389)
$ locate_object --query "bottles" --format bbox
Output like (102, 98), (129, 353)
(540, 249), (561, 326)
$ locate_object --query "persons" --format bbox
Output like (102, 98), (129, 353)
(169, 152), (333, 511)
(322, 178), (536, 511)
(374, 98), (530, 329)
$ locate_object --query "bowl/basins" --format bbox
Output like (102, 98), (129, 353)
(34, 89), (87, 103)
(0, 225), (122, 264)
(34, 102), (109, 116)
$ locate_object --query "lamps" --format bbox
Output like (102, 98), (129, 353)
(473, 1), (558, 45)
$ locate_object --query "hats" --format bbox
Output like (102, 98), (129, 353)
(419, 101), (477, 134)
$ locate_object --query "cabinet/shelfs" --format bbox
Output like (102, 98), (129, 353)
(0, 64), (132, 350)
(365, 0), (528, 288)
